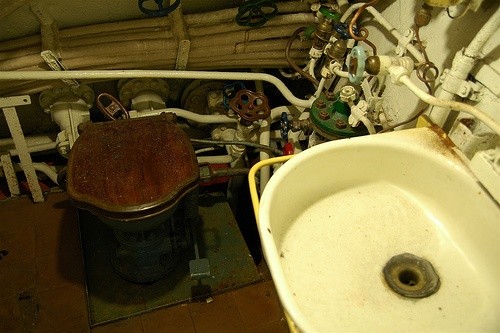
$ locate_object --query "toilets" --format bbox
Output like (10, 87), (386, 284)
(64, 112), (202, 284)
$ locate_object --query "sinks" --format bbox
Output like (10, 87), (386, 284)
(258, 126), (500, 333)
